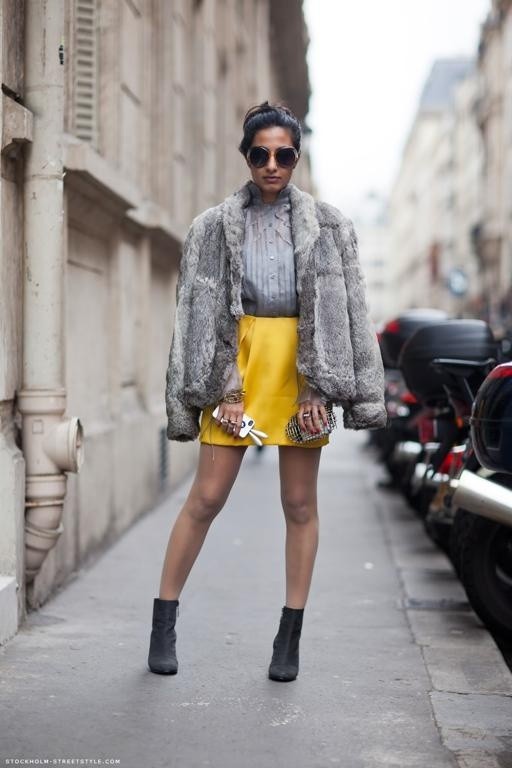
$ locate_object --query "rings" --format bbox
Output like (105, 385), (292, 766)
(221, 418), (229, 425)
(228, 420), (238, 426)
(301, 411), (312, 418)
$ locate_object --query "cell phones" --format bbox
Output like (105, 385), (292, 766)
(212, 404), (255, 438)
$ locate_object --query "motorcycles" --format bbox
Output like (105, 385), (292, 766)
(374, 308), (512, 654)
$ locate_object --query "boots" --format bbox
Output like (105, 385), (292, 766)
(149, 598), (178, 674)
(268, 606), (304, 681)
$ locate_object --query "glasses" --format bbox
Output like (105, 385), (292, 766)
(247, 145), (300, 169)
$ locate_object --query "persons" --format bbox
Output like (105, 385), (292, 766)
(146, 98), (390, 682)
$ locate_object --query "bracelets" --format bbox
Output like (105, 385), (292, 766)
(220, 388), (246, 403)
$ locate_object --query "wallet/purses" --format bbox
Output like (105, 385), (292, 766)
(286, 406), (337, 445)
